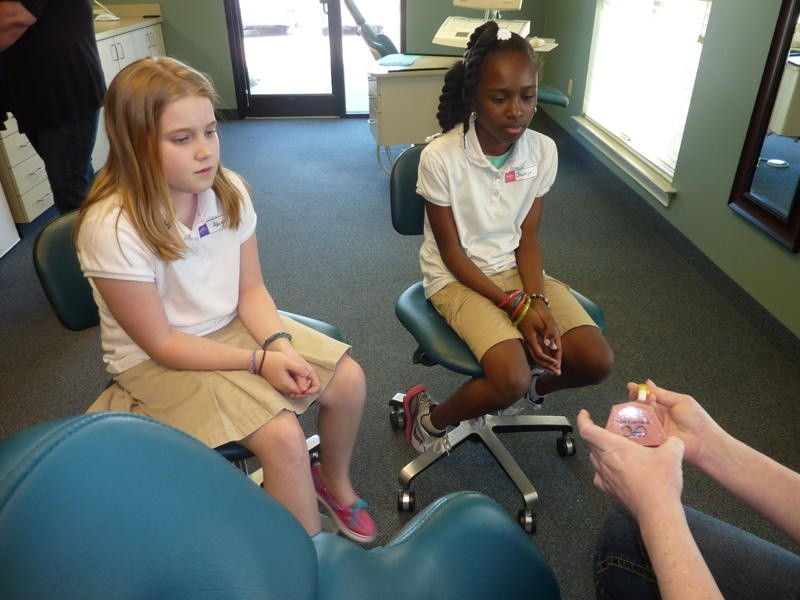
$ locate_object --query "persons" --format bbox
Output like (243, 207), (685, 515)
(70, 56), (379, 549)
(1, 1), (106, 216)
(402, 19), (615, 454)
(574, 379), (800, 600)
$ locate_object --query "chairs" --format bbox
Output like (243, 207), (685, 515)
(344, 0), (570, 109)
(34, 208), (346, 491)
(1, 411), (563, 600)
(389, 131), (610, 534)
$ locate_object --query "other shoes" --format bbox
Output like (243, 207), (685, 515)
(311, 462), (377, 543)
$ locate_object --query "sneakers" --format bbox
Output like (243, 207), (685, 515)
(403, 384), (449, 453)
(497, 367), (545, 416)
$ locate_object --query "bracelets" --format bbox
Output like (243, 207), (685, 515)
(496, 289), (532, 326)
(262, 330), (292, 351)
(251, 349), (258, 374)
(257, 349), (266, 374)
(529, 293), (551, 309)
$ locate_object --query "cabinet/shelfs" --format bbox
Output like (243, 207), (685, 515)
(90, 24), (141, 174)
(131, 16), (168, 60)
(0, 112), (55, 225)
